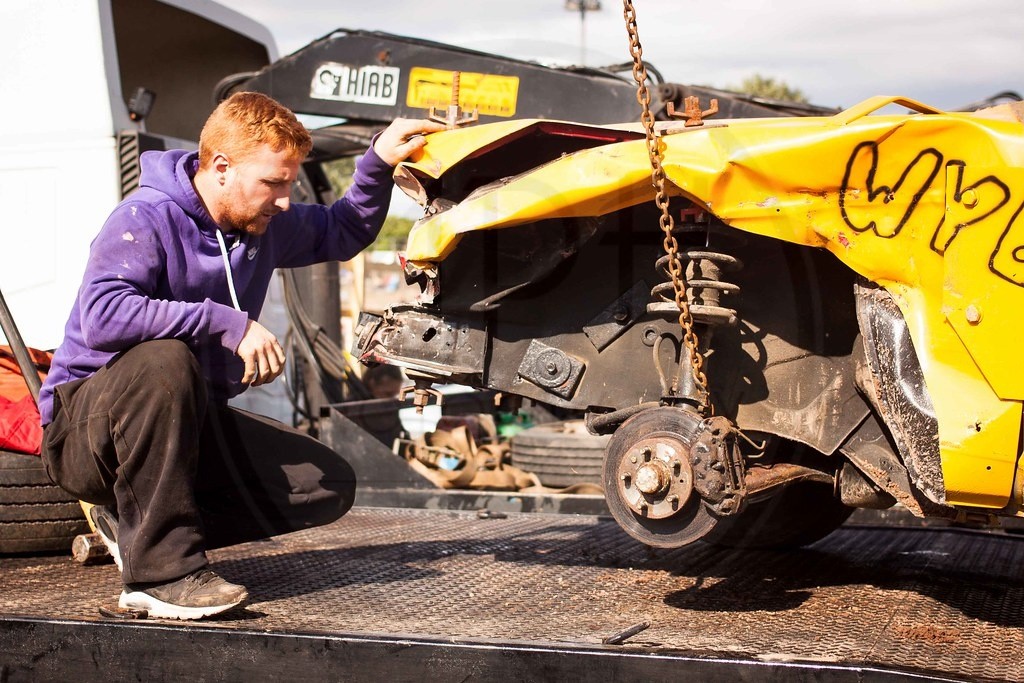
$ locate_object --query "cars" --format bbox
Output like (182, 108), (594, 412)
(349, 95), (1024, 555)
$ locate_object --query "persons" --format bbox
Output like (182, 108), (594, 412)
(37, 90), (447, 620)
(342, 362), (416, 457)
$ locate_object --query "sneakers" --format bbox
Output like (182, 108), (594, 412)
(78, 500), (124, 572)
(119, 567), (247, 619)
